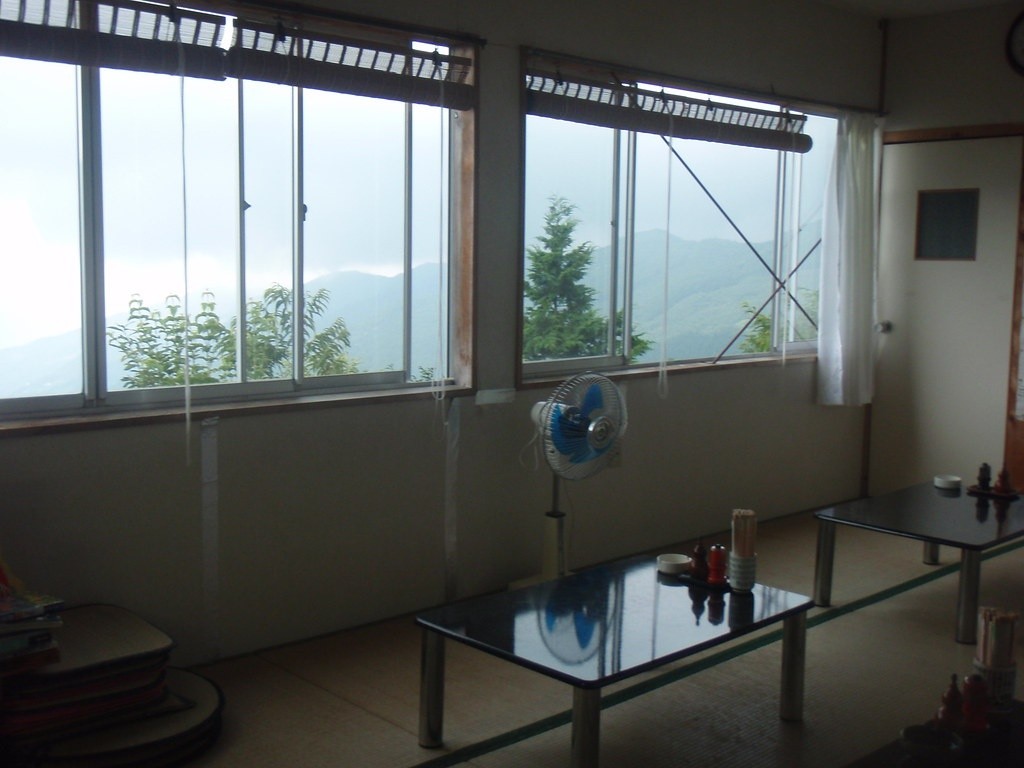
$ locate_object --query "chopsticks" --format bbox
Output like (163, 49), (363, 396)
(975, 605), (1018, 668)
(731, 509), (756, 556)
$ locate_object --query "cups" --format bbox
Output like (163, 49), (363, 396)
(971, 656), (1016, 717)
(729, 551), (757, 593)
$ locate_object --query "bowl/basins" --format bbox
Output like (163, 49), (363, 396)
(934, 474), (961, 489)
(657, 554), (692, 575)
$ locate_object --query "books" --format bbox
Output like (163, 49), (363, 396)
(0, 590), (65, 680)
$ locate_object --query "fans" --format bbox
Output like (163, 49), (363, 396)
(527, 370), (628, 576)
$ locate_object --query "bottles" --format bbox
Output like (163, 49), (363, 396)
(690, 535), (726, 583)
(978, 463), (1009, 493)
(935, 672), (987, 738)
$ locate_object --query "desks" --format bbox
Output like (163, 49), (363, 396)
(815, 480), (1024, 645)
(414, 541), (813, 768)
